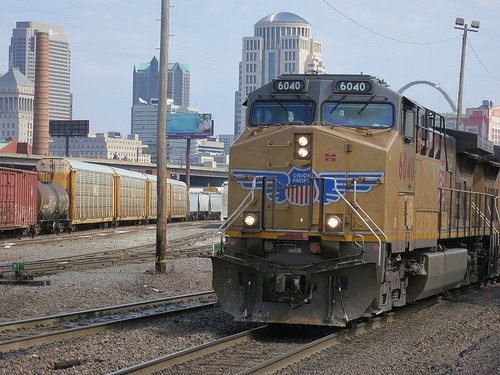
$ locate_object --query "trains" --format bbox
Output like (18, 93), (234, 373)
(0, 150), (230, 240)
(199, 70), (500, 332)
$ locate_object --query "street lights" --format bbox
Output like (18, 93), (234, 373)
(453, 17), (479, 132)
